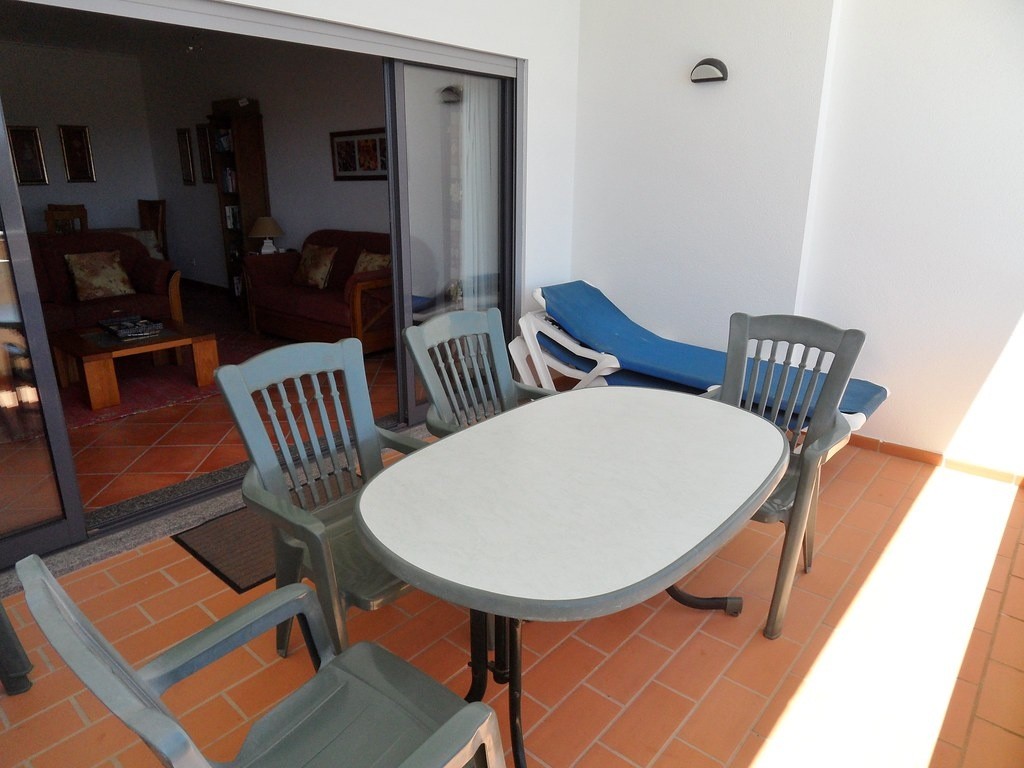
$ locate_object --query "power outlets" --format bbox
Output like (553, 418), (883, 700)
(188, 257), (195, 267)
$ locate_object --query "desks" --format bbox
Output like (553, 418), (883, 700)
(352, 384), (790, 768)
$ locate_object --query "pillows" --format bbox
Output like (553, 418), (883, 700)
(290, 244), (339, 291)
(65, 249), (137, 302)
(353, 249), (393, 296)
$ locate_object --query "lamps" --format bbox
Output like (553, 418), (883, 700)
(441, 85), (464, 105)
(689, 58), (728, 84)
(246, 217), (285, 252)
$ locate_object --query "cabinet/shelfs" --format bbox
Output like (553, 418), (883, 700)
(205, 97), (275, 287)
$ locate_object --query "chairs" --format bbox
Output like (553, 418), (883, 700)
(211, 338), (497, 658)
(508, 280), (891, 465)
(695, 311), (868, 640)
(13, 553), (507, 768)
(44, 208), (89, 234)
(137, 198), (172, 263)
(399, 308), (561, 439)
(47, 204), (85, 231)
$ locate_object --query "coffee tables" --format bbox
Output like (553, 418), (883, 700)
(47, 317), (219, 411)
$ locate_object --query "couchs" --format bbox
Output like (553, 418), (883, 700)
(28, 230), (185, 335)
(243, 229), (436, 356)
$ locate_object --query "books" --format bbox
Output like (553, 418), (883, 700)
(221, 166), (240, 192)
(98, 314), (164, 342)
(225, 205), (241, 229)
(215, 126), (234, 151)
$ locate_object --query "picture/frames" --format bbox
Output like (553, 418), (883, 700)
(7, 125), (50, 186)
(176, 127), (196, 187)
(57, 124), (97, 183)
(196, 123), (216, 183)
(329, 126), (388, 181)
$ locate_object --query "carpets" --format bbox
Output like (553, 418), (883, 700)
(170, 468), (364, 596)
